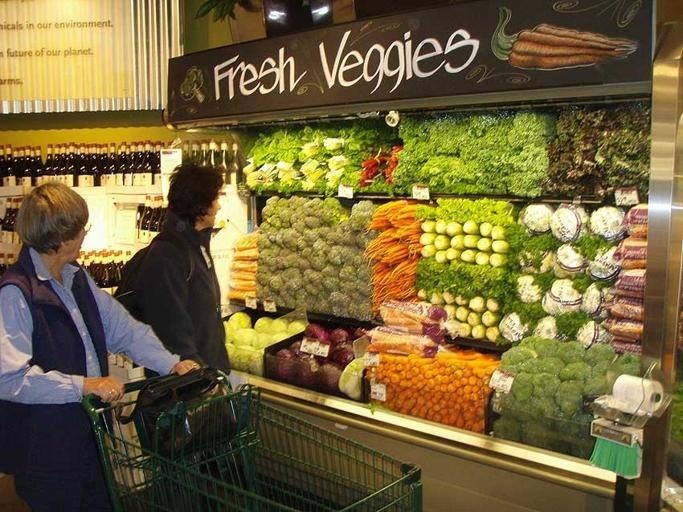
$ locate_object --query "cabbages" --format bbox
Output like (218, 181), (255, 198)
(498, 205), (625, 349)
(222, 311), (310, 376)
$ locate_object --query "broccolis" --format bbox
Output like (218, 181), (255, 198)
(494, 336), (642, 460)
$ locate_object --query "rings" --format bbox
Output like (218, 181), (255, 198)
(191, 363), (198, 369)
(110, 388), (115, 393)
(106, 390), (112, 395)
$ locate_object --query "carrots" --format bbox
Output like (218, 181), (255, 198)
(364, 200), (501, 434)
(508, 24), (639, 67)
(228, 234), (259, 301)
(610, 224), (648, 340)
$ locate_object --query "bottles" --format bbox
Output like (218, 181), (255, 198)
(131, 195), (169, 243)
(0, 249), (130, 295)
(1, 197), (25, 246)
(0, 139), (240, 188)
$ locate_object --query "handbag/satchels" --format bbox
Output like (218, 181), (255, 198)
(111, 232), (195, 322)
(118, 369), (241, 457)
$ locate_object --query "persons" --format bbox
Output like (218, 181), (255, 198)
(131, 159), (234, 511)
(0, 181), (206, 511)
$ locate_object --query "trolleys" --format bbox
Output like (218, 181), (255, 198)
(84, 371), (423, 512)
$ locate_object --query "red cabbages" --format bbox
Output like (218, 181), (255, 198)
(274, 323), (371, 401)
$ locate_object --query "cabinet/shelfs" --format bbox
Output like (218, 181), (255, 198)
(0, 81), (682, 509)
